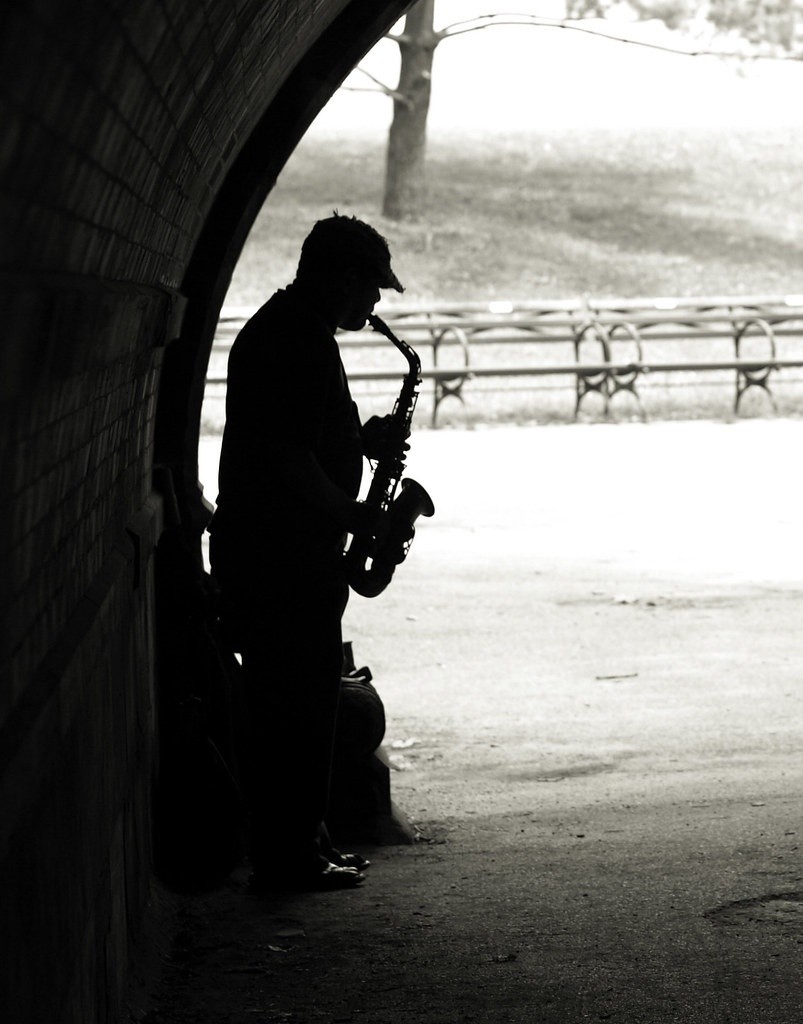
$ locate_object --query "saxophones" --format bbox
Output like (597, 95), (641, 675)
(343, 313), (435, 599)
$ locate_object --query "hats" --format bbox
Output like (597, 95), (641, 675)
(305, 215), (404, 294)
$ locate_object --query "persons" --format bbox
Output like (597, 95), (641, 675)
(217, 216), (412, 890)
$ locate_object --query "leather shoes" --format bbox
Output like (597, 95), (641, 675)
(296, 850), (372, 889)
